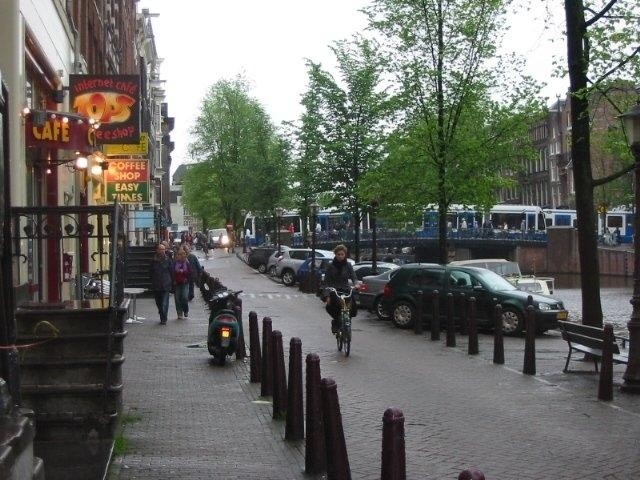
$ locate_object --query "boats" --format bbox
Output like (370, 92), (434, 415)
(449, 256), (555, 304)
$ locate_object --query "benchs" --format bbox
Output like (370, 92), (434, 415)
(557, 317), (633, 373)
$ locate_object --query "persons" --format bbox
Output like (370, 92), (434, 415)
(323, 244), (361, 320)
(201, 212), (636, 260)
(148, 230), (201, 324)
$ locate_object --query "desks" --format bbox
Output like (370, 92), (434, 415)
(124, 288), (148, 324)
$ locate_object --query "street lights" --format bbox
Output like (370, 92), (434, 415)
(306, 201), (320, 293)
(240, 207), (248, 254)
(275, 204), (283, 261)
(618, 94), (640, 393)
(369, 202), (381, 277)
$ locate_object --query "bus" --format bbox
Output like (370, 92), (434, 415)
(423, 201), (546, 241)
(239, 206), (358, 241)
(340, 201), (413, 235)
(543, 207), (634, 244)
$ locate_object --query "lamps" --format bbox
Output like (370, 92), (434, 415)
(19, 107), (103, 179)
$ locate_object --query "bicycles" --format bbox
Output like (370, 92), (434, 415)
(325, 283), (358, 357)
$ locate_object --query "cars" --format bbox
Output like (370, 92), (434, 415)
(206, 228), (229, 249)
(169, 230), (203, 250)
(267, 248), (297, 278)
(321, 264), (401, 303)
(385, 264), (570, 335)
(297, 256), (356, 293)
(249, 244), (293, 274)
(360, 263), (446, 320)
(276, 248), (356, 286)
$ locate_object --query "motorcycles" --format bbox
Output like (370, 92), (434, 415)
(203, 281), (244, 366)
